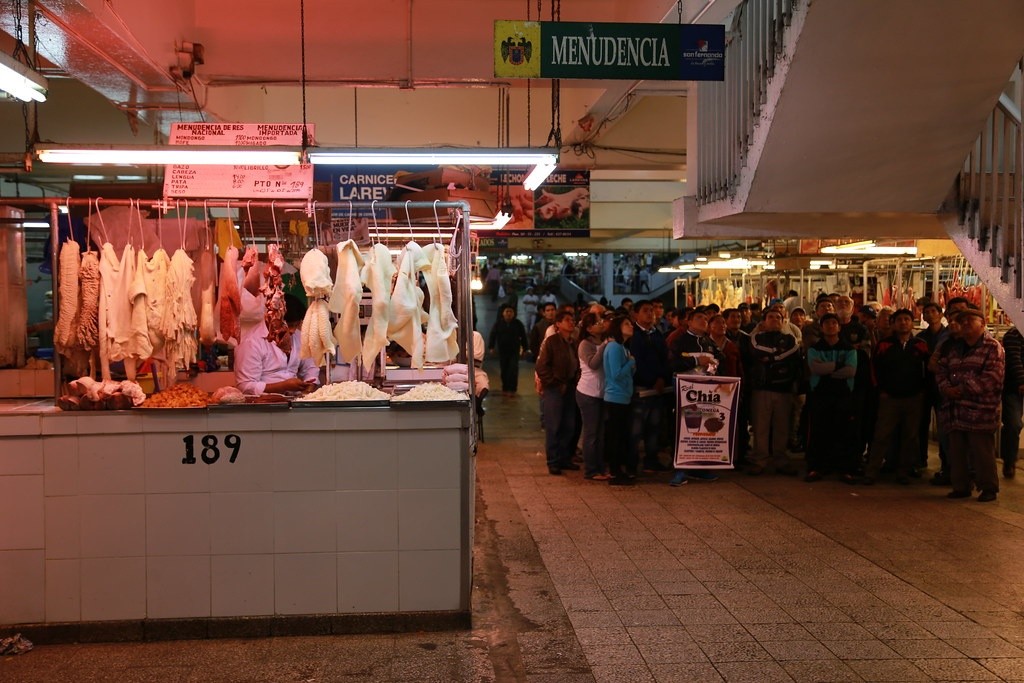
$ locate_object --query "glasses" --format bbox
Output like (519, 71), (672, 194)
(594, 319), (604, 327)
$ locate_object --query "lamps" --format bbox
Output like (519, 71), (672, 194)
(10, 1), (563, 238)
(0, 0), (49, 103)
(655, 229), (919, 272)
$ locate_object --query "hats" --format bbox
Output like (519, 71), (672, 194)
(858, 305), (877, 320)
(955, 310), (985, 324)
(790, 306), (807, 317)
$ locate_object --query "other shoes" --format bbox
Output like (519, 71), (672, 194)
(540, 444), (1016, 503)
(502, 392), (516, 397)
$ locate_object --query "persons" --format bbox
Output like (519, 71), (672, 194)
(487, 290), (981, 488)
(933, 308), (1006, 502)
(234, 293), (321, 396)
(1000, 326), (1024, 481)
(481, 253), (650, 337)
